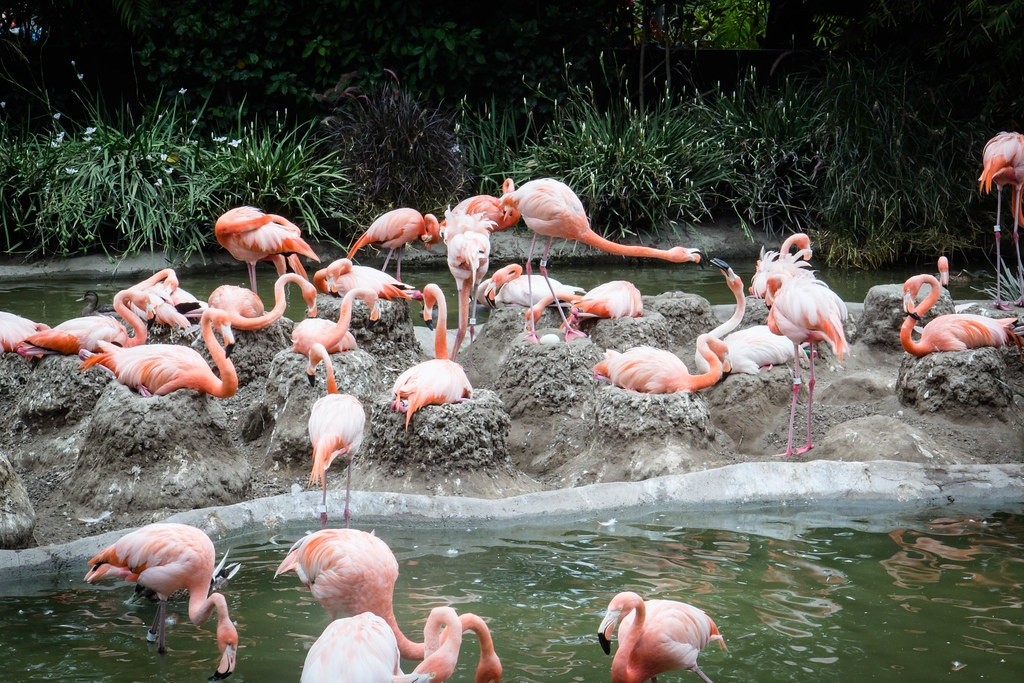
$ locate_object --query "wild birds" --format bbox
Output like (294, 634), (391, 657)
(0, 130), (1024, 683)
